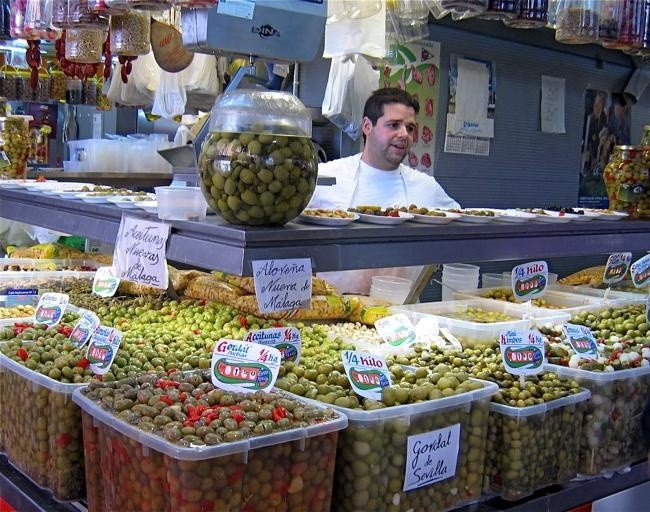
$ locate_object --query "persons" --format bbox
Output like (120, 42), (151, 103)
(584, 95), (630, 163)
(311, 88), (460, 294)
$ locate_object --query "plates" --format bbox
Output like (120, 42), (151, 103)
(1, 176), (158, 215)
(299, 207), (630, 227)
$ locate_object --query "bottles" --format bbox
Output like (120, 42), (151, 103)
(547, 0), (625, 50)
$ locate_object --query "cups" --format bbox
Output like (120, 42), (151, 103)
(326, 0), (448, 45)
(150, 133), (168, 148)
(370, 277), (410, 306)
(481, 270), (557, 291)
(441, 261), (480, 299)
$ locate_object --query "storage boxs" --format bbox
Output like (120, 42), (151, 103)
(400, 348), (592, 503)
(309, 362), (501, 512)
(70, 371), (349, 511)
(542, 351), (649, 478)
(0, 257), (106, 511)
(389, 283), (648, 343)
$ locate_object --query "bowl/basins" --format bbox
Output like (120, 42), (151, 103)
(154, 186), (210, 218)
